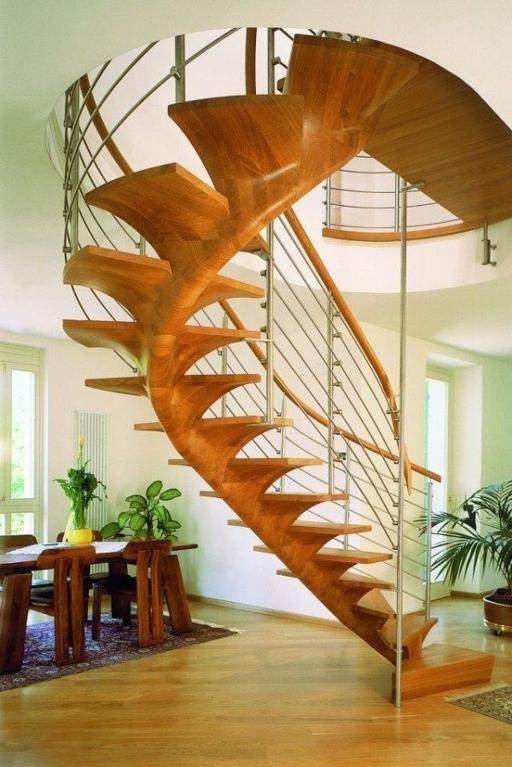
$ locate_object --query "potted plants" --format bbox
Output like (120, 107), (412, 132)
(51, 436), (104, 528)
(413, 481), (511, 636)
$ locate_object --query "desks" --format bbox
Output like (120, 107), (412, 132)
(0, 541), (205, 674)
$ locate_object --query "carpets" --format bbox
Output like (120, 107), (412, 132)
(447, 678), (511, 732)
(1, 584), (237, 695)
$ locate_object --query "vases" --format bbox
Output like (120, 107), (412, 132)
(63, 529), (93, 544)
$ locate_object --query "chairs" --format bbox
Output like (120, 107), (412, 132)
(35, 551), (93, 664)
(90, 535), (171, 646)
(0, 525), (41, 554)
(57, 530), (104, 545)
